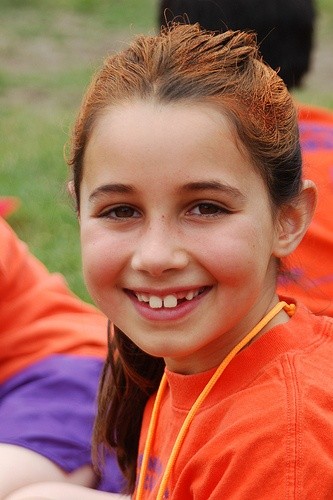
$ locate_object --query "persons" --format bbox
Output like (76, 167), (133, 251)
(1, 18), (332, 500)
(155, 2), (332, 316)
(2, 196), (130, 500)
